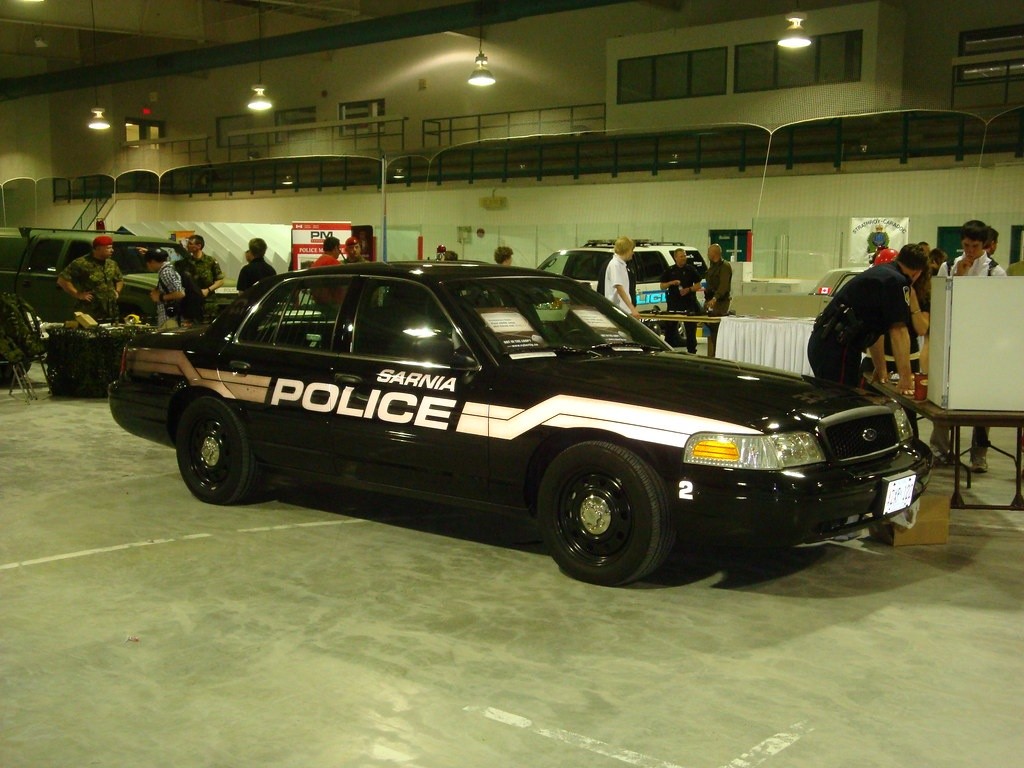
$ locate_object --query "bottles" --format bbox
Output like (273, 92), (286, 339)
(678, 284), (684, 296)
(166, 301), (177, 320)
(700, 276), (707, 290)
(915, 367), (928, 400)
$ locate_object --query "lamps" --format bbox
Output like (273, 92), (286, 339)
(777, 0), (812, 49)
(393, 169), (405, 178)
(282, 176), (293, 184)
(247, 0), (272, 110)
(468, 0), (496, 86)
(88, 0), (111, 130)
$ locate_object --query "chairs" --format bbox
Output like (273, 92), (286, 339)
(0, 355), (52, 405)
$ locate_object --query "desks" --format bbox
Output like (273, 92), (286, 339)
(865, 372), (1024, 509)
(47, 326), (210, 397)
(637, 312), (816, 379)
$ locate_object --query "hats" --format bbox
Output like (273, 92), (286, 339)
(345, 236), (358, 248)
(92, 236), (113, 247)
(143, 248), (169, 263)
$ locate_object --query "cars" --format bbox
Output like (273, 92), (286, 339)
(108, 261), (936, 587)
(811, 267), (867, 296)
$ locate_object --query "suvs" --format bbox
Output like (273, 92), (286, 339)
(0, 227), (241, 388)
(535, 238), (709, 346)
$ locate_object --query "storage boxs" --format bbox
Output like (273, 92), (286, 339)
(927, 276), (1024, 412)
(869, 499), (951, 545)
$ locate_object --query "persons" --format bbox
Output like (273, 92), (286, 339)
(494, 246), (513, 265)
(144, 248), (185, 326)
(597, 237), (640, 318)
(236, 238), (277, 295)
(807, 220), (1006, 472)
(659, 248), (702, 353)
(183, 235), (224, 322)
(704, 244), (733, 355)
(57, 236), (123, 398)
(310, 236), (372, 304)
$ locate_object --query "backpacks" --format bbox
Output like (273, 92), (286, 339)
(158, 272), (207, 323)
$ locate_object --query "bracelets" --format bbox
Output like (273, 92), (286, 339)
(160, 294), (165, 301)
(207, 287), (211, 292)
(116, 291), (120, 295)
(911, 310), (921, 314)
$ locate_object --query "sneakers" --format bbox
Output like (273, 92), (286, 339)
(930, 443), (955, 465)
(972, 455), (988, 472)
